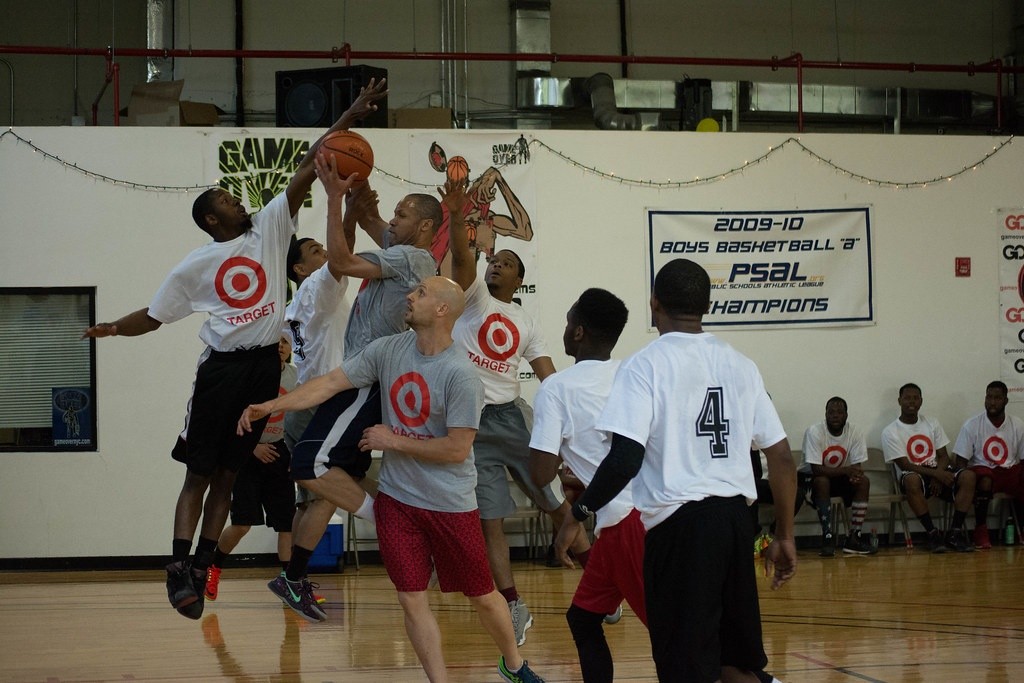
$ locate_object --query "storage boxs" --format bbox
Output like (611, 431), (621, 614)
(127, 79), (184, 129)
(179, 99), (226, 128)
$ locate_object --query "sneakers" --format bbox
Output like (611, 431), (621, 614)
(973, 525), (992, 549)
(166, 556), (203, 620)
(945, 531), (974, 552)
(842, 538), (876, 555)
(189, 565), (208, 614)
(929, 533), (946, 554)
(819, 541), (835, 557)
(509, 597), (534, 648)
(498, 652), (545, 683)
(282, 592), (325, 609)
(603, 603), (622, 624)
(267, 571), (329, 623)
(203, 563), (221, 601)
(754, 532), (773, 557)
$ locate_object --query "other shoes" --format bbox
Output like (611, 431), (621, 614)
(545, 557), (562, 569)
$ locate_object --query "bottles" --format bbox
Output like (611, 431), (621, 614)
(869, 528), (878, 551)
(1004, 517), (1014, 545)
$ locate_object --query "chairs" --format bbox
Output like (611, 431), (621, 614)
(790, 447), (1024, 549)
(502, 480), (541, 561)
(347, 457), (382, 570)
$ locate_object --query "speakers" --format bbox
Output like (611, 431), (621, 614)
(276, 65), (388, 128)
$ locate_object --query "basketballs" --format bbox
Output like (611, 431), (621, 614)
(318, 130), (375, 190)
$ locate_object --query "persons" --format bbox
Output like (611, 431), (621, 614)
(527, 289), (647, 683)
(83, 75), (621, 649)
(950, 380), (1024, 549)
(797, 396), (877, 559)
(556, 259), (797, 683)
(882, 383), (977, 553)
(236, 277), (548, 683)
